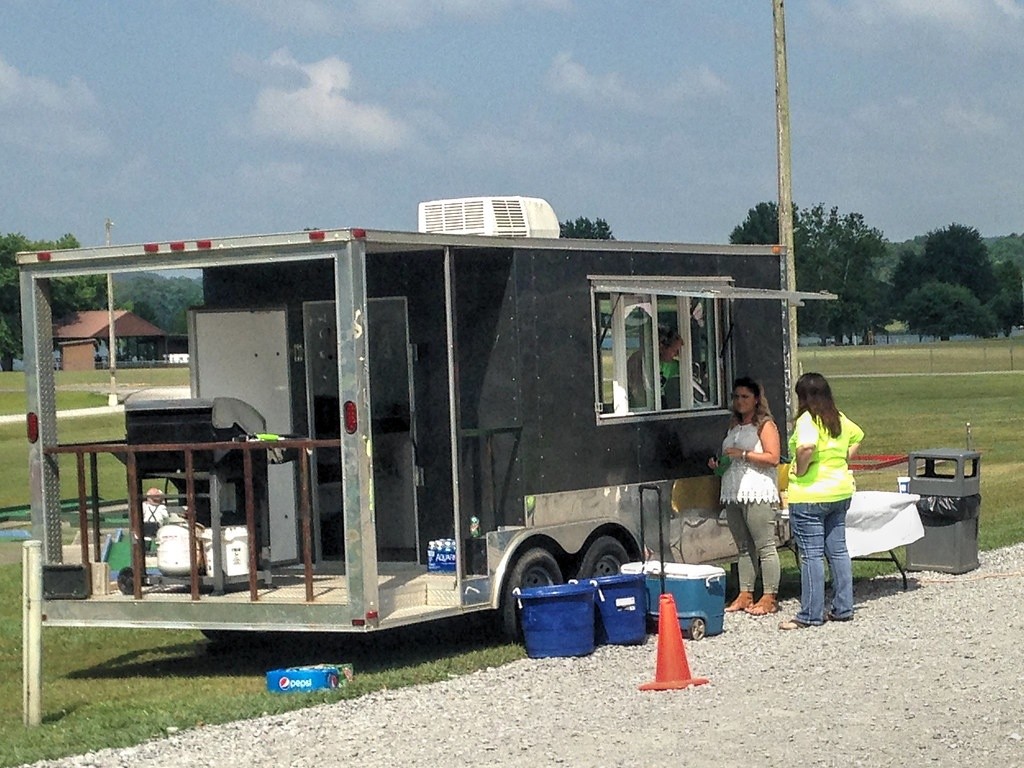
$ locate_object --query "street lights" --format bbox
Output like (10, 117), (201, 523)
(105, 218), (118, 407)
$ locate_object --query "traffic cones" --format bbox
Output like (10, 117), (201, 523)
(637, 592), (709, 691)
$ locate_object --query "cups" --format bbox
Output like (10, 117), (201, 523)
(897, 477), (912, 493)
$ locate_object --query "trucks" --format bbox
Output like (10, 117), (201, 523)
(10, 193), (842, 648)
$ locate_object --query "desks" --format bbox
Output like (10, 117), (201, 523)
(780, 492), (925, 594)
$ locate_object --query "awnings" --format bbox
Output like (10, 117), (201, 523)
(594, 284), (837, 354)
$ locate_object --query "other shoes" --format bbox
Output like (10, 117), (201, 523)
(724, 591), (754, 611)
(746, 593), (778, 615)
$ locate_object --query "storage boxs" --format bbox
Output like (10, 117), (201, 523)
(266, 662), (356, 694)
(621, 561), (727, 642)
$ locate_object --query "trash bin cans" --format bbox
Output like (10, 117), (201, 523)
(904, 448), (979, 575)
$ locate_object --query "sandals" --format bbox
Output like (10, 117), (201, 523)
(825, 611), (854, 621)
(779, 619), (808, 630)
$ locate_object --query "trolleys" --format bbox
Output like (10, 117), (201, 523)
(110, 396), (308, 596)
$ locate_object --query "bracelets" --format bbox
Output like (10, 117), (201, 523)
(742, 449), (746, 462)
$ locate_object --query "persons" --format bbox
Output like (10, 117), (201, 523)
(627, 333), (683, 406)
(708, 378), (781, 616)
(779, 372), (864, 629)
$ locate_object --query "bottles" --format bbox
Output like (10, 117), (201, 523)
(714, 454), (732, 476)
(427, 538), (456, 573)
(470, 515), (480, 537)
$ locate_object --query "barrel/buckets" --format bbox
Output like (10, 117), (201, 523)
(568, 573), (648, 647)
(511, 585), (596, 659)
